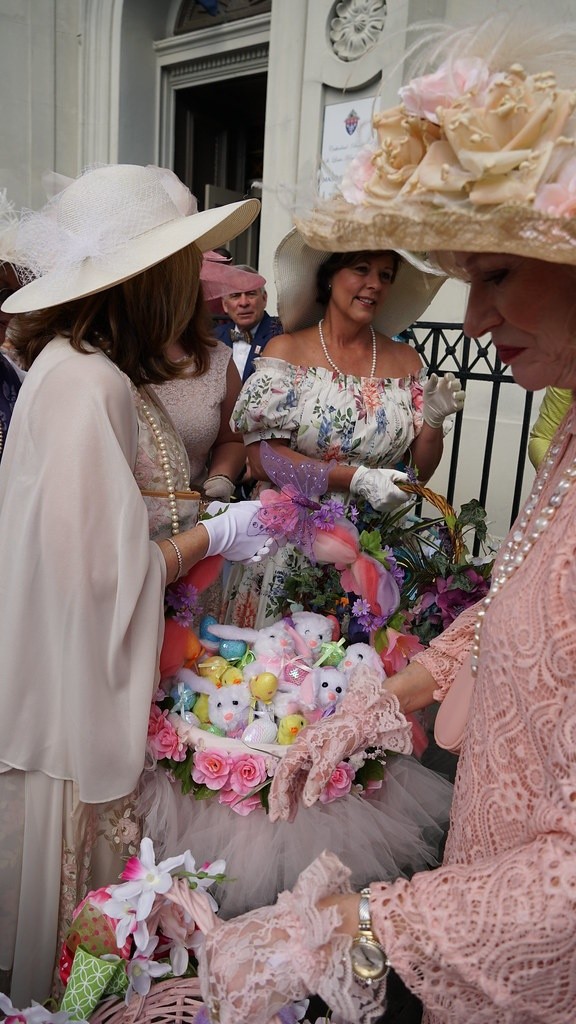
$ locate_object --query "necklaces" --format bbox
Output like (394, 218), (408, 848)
(133, 382), (194, 535)
(318, 319), (378, 378)
(471, 417), (576, 672)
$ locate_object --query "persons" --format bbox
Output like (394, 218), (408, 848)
(204, 197), (468, 631)
(1, 160), (287, 1014)
(198, 9), (576, 1024)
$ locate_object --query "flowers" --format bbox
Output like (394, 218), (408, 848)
(337, 55), (576, 219)
(0, 836), (236, 1024)
(147, 466), (505, 819)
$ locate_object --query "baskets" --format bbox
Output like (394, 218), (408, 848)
(282, 482), (463, 621)
(81, 876), (298, 1024)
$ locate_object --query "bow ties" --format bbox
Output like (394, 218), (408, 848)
(229, 329), (251, 344)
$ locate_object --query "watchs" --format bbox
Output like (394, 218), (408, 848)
(346, 888), (390, 984)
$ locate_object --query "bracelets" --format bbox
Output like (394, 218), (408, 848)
(209, 474), (235, 486)
(166, 538), (182, 582)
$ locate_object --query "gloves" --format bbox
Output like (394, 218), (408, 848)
(349, 466), (414, 514)
(203, 501), (287, 565)
(422, 371), (466, 430)
(196, 502), (286, 562)
(203, 476), (236, 502)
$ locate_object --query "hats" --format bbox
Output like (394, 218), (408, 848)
(277, 19), (576, 266)
(0, 164), (261, 314)
(273, 223), (448, 342)
